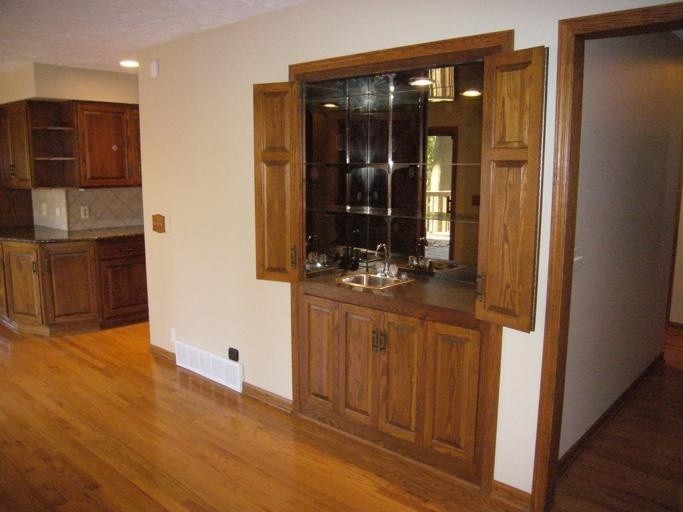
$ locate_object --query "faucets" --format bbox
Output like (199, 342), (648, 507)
(416, 236), (429, 268)
(374, 242), (391, 276)
(309, 234), (319, 263)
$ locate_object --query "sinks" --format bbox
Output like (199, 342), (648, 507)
(342, 272), (402, 288)
(305, 261), (322, 271)
(431, 259), (458, 271)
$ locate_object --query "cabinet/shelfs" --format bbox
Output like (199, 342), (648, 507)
(26, 100), (82, 191)
(0, 239), (44, 335)
(250, 27), (551, 509)
(76, 100), (142, 189)
(0, 99), (32, 191)
(40, 242), (100, 337)
(96, 235), (149, 330)
